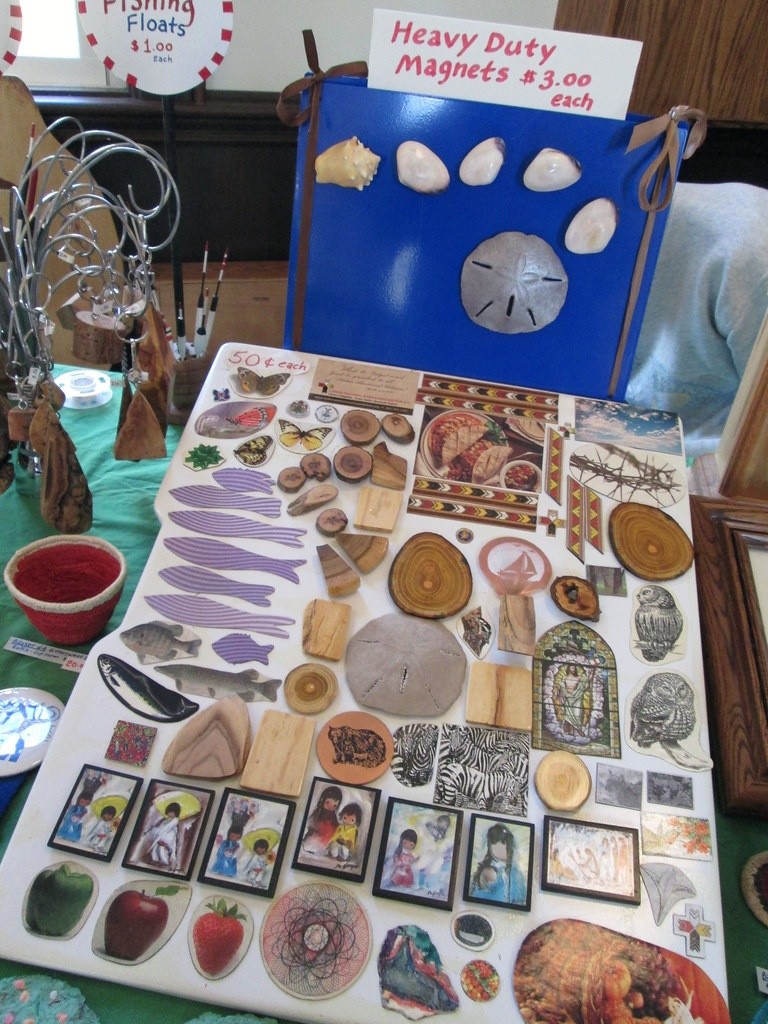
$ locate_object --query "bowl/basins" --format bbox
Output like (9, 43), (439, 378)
(3, 534), (127, 646)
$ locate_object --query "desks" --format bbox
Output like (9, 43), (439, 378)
(0, 363), (768, 1024)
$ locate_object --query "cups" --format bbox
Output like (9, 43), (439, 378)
(166, 351), (209, 409)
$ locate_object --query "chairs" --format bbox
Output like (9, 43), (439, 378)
(0, 76), (124, 370)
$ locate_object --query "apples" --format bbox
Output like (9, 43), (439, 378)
(103, 888), (169, 961)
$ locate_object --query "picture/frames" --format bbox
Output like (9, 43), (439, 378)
(688, 495), (768, 822)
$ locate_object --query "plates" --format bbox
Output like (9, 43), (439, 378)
(0, 687), (66, 776)
(419, 409), (509, 484)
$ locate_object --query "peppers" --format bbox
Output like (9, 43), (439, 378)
(25, 864), (93, 936)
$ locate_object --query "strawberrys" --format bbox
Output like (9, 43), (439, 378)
(193, 898), (248, 975)
